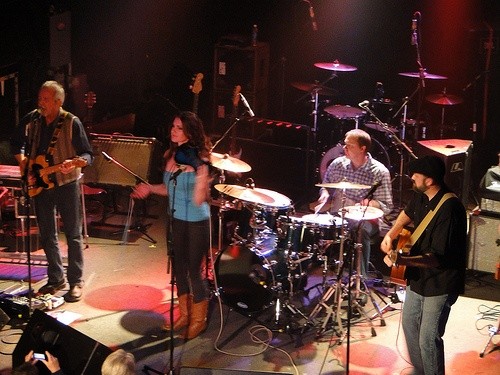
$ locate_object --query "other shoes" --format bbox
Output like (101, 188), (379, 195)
(360, 293), (367, 304)
(68, 280), (83, 302)
(39, 278), (66, 295)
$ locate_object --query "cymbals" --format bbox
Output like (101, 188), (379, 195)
(398, 72), (448, 79)
(292, 82), (344, 96)
(313, 62), (358, 71)
(214, 183), (291, 207)
(338, 205), (383, 220)
(315, 181), (372, 190)
(210, 152), (252, 173)
(425, 93), (465, 105)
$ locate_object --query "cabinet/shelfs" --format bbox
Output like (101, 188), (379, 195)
(211, 40), (271, 140)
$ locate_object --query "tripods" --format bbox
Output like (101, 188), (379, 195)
(391, 80), (424, 191)
(272, 195), (398, 343)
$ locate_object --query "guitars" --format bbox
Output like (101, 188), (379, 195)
(20, 156), (88, 198)
(84, 91), (97, 133)
(224, 83), (242, 182)
(383, 229), (411, 286)
(189, 72), (204, 113)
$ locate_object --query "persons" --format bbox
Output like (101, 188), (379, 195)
(100, 348), (136, 375)
(314, 128), (395, 305)
(16, 80), (94, 302)
(381, 154), (469, 375)
(15, 349), (66, 375)
(0, 134), (11, 162)
(128, 110), (213, 341)
(34, 62), (89, 111)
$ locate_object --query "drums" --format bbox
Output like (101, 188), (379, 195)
(364, 95), (401, 126)
(300, 215), (352, 264)
(252, 204), (294, 239)
(400, 120), (428, 144)
(363, 122), (400, 145)
(317, 105), (366, 149)
(316, 131), (401, 183)
(214, 233), (308, 314)
(277, 214), (321, 256)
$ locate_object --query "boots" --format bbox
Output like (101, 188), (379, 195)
(161, 293), (189, 331)
(180, 298), (208, 340)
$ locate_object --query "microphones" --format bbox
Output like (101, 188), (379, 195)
(309, 6), (318, 31)
(359, 100), (370, 107)
(363, 179), (382, 199)
(411, 19), (418, 45)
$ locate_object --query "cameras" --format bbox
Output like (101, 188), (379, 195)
(32, 351), (48, 361)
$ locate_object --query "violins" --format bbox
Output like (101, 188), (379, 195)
(175, 139), (224, 177)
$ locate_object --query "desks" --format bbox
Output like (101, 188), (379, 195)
(83, 182), (160, 230)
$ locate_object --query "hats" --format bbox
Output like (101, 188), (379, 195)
(407, 155), (446, 181)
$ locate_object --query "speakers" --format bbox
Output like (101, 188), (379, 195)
(468, 214), (500, 273)
(212, 89), (268, 136)
(38, 11), (70, 67)
(415, 139), (474, 197)
(82, 140), (152, 187)
(215, 40), (270, 91)
(12, 301), (180, 375)
